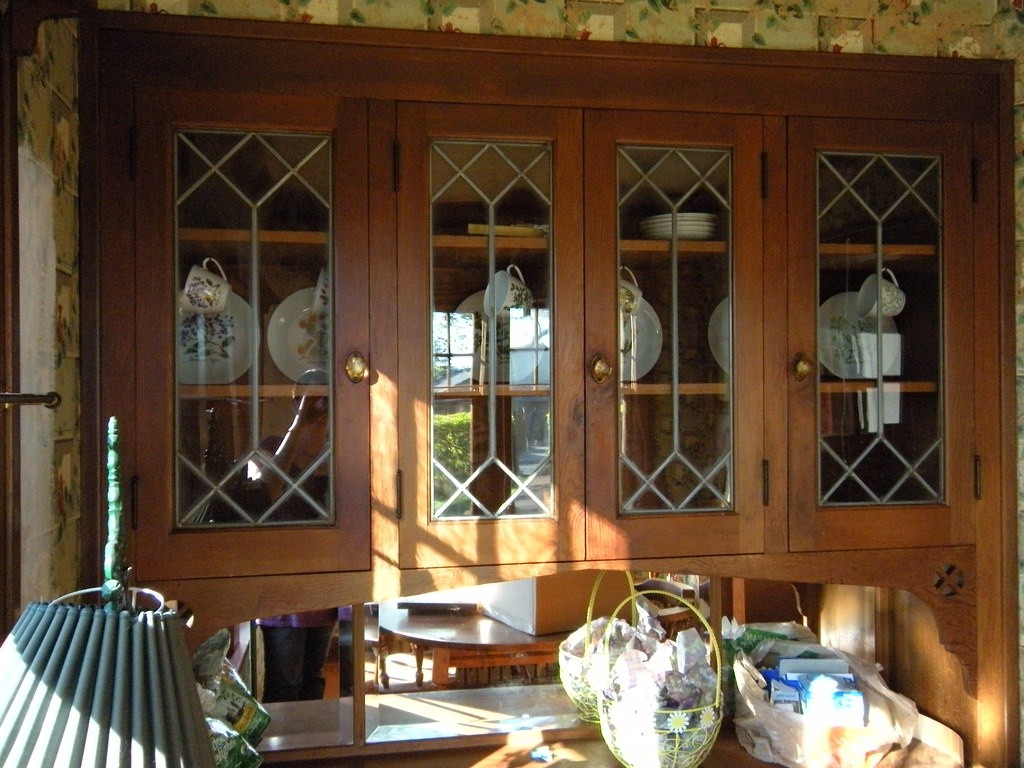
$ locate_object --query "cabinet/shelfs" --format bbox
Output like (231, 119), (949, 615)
(76, 8), (1015, 767)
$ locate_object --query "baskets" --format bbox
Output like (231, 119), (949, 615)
(594, 591), (725, 768)
(560, 569), (638, 724)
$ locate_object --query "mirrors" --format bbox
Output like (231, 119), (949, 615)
(225, 568), (876, 753)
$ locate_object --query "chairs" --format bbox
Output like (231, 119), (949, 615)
(634, 577), (694, 644)
(431, 648), (564, 691)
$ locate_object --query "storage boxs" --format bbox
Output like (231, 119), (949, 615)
(477, 569), (633, 636)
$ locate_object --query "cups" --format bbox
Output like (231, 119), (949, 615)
(181, 256), (231, 314)
(856, 268), (906, 317)
(311, 269), (332, 316)
(618, 265), (643, 316)
(483, 265), (533, 319)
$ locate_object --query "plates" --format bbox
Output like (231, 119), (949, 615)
(177, 288), (255, 385)
(450, 289), (545, 382)
(708, 297), (730, 372)
(617, 297), (663, 382)
(268, 284), (332, 385)
(820, 291), (899, 380)
(641, 211), (718, 240)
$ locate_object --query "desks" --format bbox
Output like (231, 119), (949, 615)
(377, 586), (578, 650)
